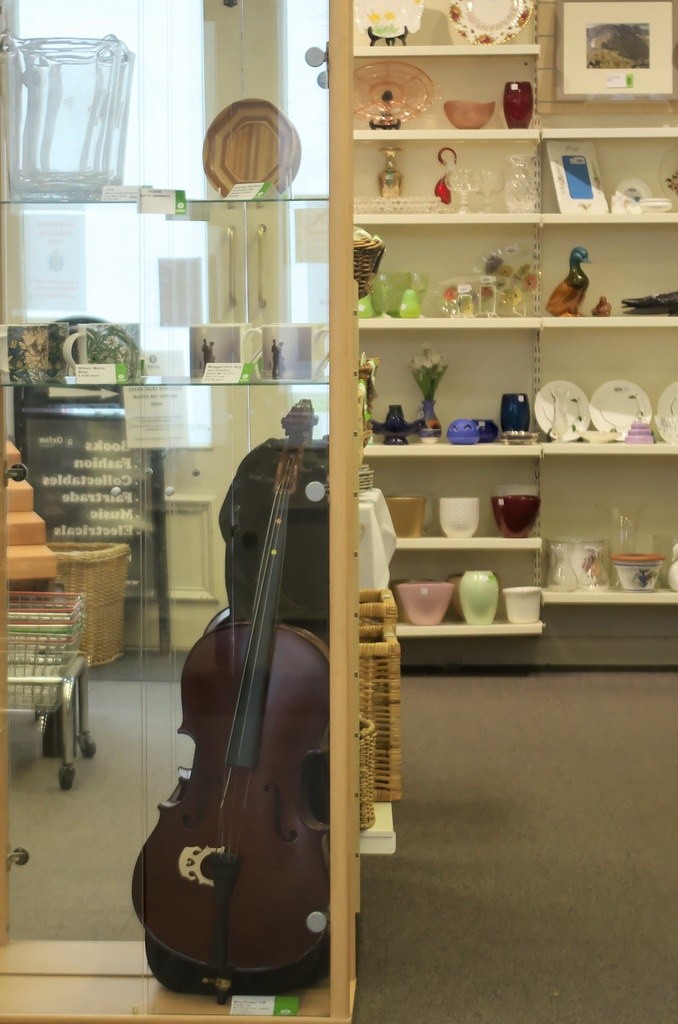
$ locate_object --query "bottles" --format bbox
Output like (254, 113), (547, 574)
(379, 148), (402, 199)
(505, 154), (537, 213)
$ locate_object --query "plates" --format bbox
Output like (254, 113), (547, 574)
(203, 98), (294, 200)
(590, 380), (652, 441)
(655, 381), (678, 445)
(277, 119), (302, 194)
(535, 381), (590, 441)
(354, 61), (434, 124)
(354, 0), (424, 38)
(449, 0), (533, 46)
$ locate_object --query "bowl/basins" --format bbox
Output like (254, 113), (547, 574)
(578, 431), (621, 443)
(445, 101), (495, 129)
(397, 583), (455, 625)
(640, 199), (673, 213)
(439, 498), (479, 538)
(492, 495), (541, 538)
(654, 414), (678, 445)
(611, 554), (666, 591)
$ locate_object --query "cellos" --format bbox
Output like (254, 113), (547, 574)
(130, 398), (331, 1005)
(166, 400), (318, 806)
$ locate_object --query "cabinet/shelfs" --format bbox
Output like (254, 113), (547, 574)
(349, 2), (543, 636)
(541, 127), (677, 607)
(0, 0), (350, 1024)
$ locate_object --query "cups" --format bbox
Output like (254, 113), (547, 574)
(501, 394), (529, 432)
(0, 324), (48, 383)
(272, 323), (329, 379)
(48, 323), (69, 382)
(62, 323), (131, 384)
(262, 323), (330, 381)
(128, 323), (141, 385)
(190, 324), (262, 379)
(503, 82), (532, 128)
(245, 324), (263, 379)
(504, 587), (541, 624)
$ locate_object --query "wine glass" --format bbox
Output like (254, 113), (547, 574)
(475, 169), (499, 214)
(449, 169), (479, 213)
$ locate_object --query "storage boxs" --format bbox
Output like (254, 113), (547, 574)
(359, 715), (375, 830)
(358, 585), (398, 633)
(357, 625), (403, 802)
(554, 1), (676, 103)
(45, 541), (130, 669)
